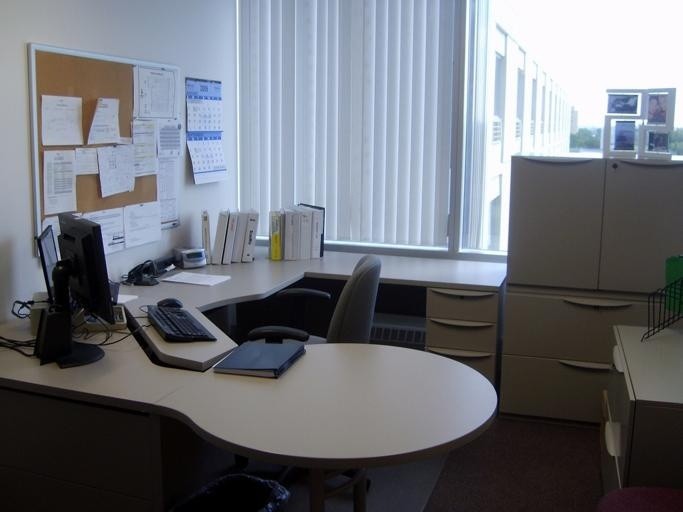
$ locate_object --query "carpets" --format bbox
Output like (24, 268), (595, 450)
(203, 449), (454, 511)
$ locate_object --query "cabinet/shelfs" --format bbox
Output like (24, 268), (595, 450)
(498, 154), (683, 496)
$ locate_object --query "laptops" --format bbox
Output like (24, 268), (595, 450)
(37, 224), (120, 305)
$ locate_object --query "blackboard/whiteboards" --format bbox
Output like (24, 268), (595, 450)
(30, 44), (187, 259)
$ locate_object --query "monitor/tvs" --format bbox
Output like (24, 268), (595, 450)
(32, 214), (116, 368)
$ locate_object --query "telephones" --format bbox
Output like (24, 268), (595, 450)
(128, 260), (158, 286)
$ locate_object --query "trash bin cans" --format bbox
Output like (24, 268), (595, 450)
(167, 473), (292, 512)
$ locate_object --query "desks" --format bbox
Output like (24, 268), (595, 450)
(1, 245), (507, 512)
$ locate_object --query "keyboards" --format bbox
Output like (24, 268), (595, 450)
(146, 305), (218, 342)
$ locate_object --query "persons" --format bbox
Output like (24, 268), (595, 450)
(650, 134), (667, 152)
(647, 94), (666, 124)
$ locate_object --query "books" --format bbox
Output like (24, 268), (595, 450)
(213, 341), (306, 379)
(268, 203), (325, 261)
(201, 209), (259, 265)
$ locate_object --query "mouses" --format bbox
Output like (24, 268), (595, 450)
(156, 298), (183, 308)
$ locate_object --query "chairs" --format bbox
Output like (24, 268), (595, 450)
(243, 253), (387, 494)
